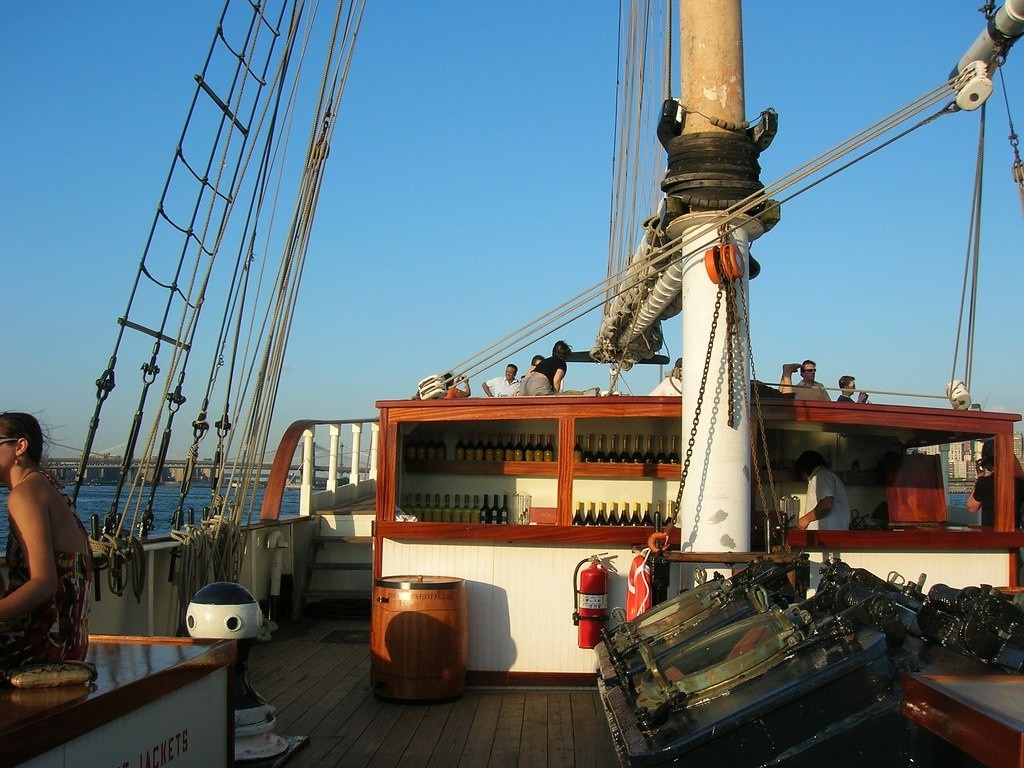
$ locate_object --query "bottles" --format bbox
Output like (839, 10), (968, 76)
(404, 432), (554, 463)
(572, 501), (584, 526)
(574, 433), (679, 465)
(402, 492), (507, 525)
(641, 500), (674, 526)
(584, 501), (594, 527)
(606, 502), (618, 526)
(595, 501), (606, 526)
(618, 502), (630, 526)
(629, 501), (641, 526)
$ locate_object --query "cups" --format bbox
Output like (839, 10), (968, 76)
(513, 495), (530, 525)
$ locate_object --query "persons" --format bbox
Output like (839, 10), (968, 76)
(444, 373), (471, 398)
(482, 364), (525, 397)
(793, 360), (831, 401)
(837, 376), (868, 403)
(967, 437), (1024, 589)
(649, 358), (804, 399)
(520, 341), (572, 396)
(526, 355), (545, 375)
(795, 450), (850, 530)
(0, 412), (92, 686)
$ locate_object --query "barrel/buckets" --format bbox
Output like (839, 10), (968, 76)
(370, 573), (468, 702)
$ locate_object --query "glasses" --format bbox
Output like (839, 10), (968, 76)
(804, 368), (817, 373)
(848, 384), (856, 387)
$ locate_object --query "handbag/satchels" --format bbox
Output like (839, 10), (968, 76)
(6, 659), (98, 689)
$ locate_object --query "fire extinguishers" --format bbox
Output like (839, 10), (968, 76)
(573, 553), (607, 649)
